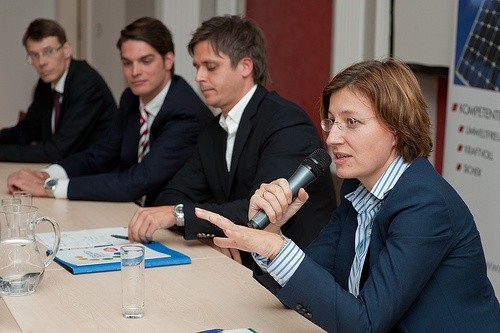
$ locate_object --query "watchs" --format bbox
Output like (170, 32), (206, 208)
(43, 177), (58, 198)
(172, 203), (185, 226)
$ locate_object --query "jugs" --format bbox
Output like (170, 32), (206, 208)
(0, 205), (61, 296)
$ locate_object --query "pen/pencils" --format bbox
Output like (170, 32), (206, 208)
(111, 235), (151, 244)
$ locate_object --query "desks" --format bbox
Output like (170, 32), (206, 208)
(0, 162), (327, 333)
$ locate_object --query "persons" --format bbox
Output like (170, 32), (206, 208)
(194, 58), (500, 333)
(6, 17), (214, 208)
(126, 15), (337, 270)
(0, 18), (118, 163)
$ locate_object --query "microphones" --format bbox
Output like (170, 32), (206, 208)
(247, 148), (332, 231)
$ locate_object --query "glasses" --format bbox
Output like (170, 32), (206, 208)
(321, 116), (378, 133)
(24, 45), (62, 64)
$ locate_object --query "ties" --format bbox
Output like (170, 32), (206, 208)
(53, 88), (62, 132)
(138, 108), (151, 165)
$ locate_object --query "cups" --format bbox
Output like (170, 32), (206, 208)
(1, 191), (34, 240)
(120, 245), (145, 319)
